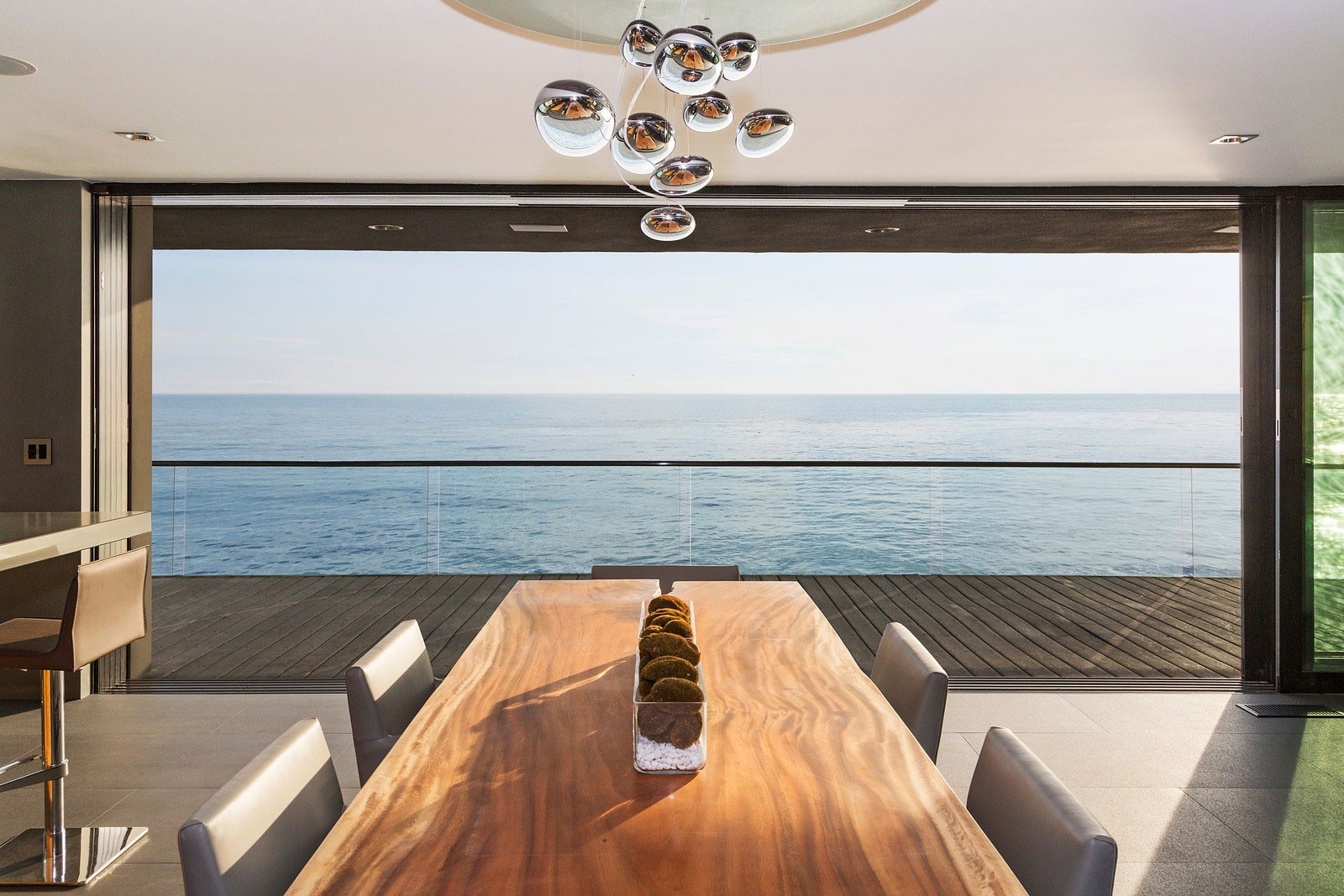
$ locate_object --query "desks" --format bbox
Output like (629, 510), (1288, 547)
(284, 580), (1029, 896)
(0, 512), (152, 570)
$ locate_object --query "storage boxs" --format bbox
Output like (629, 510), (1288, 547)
(633, 600), (707, 775)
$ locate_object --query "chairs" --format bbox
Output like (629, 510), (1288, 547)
(965, 726), (1118, 896)
(591, 564), (738, 580)
(180, 718), (347, 896)
(870, 621), (949, 766)
(345, 618), (442, 790)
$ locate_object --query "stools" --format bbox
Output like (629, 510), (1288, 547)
(0, 543), (150, 886)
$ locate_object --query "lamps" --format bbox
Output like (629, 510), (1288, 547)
(535, 0), (795, 241)
